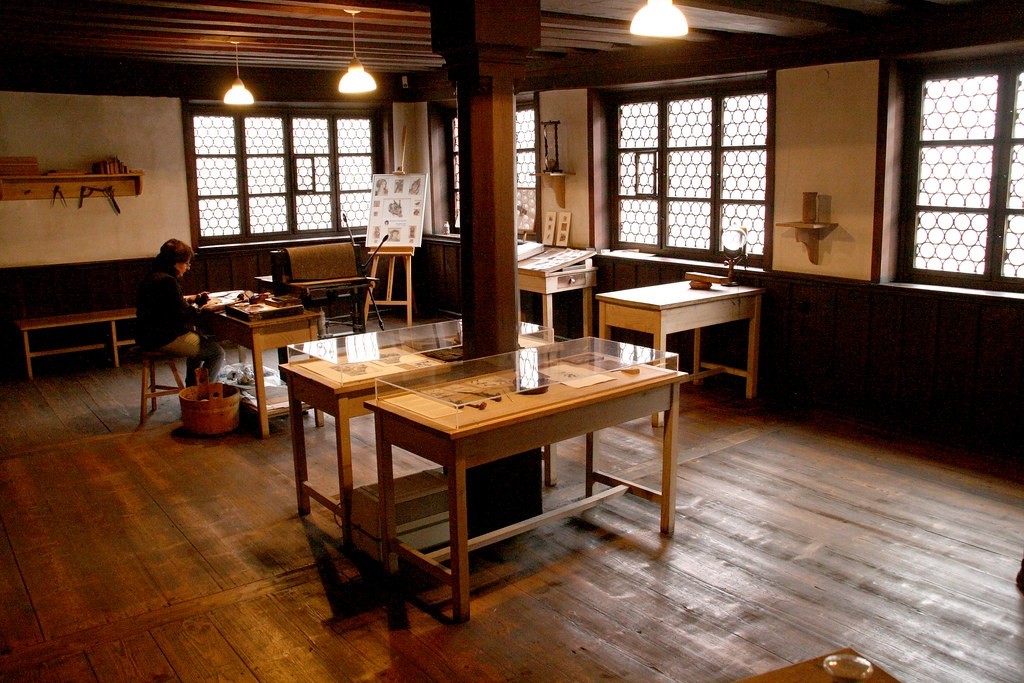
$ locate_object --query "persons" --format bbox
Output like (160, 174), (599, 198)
(135, 239), (226, 387)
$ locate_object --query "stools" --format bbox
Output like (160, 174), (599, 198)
(127, 344), (185, 425)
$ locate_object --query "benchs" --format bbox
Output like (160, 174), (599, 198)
(15, 305), (138, 380)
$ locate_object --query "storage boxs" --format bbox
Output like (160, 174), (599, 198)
(350, 470), (465, 563)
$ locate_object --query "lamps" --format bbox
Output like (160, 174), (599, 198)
(720, 226), (747, 286)
(628, 0), (690, 37)
(338, 8), (378, 96)
(223, 39), (256, 107)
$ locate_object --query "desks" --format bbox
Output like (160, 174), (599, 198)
(279, 334), (556, 554)
(595, 277), (766, 428)
(362, 348), (689, 620)
(736, 646), (901, 683)
(517, 241), (598, 341)
(253, 244), (380, 381)
(184, 289), (324, 442)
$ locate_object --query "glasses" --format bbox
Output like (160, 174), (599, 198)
(184, 262), (192, 267)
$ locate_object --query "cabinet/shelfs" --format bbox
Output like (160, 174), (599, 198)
(1, 170), (149, 205)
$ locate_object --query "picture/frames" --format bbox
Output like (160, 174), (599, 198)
(365, 171), (429, 247)
